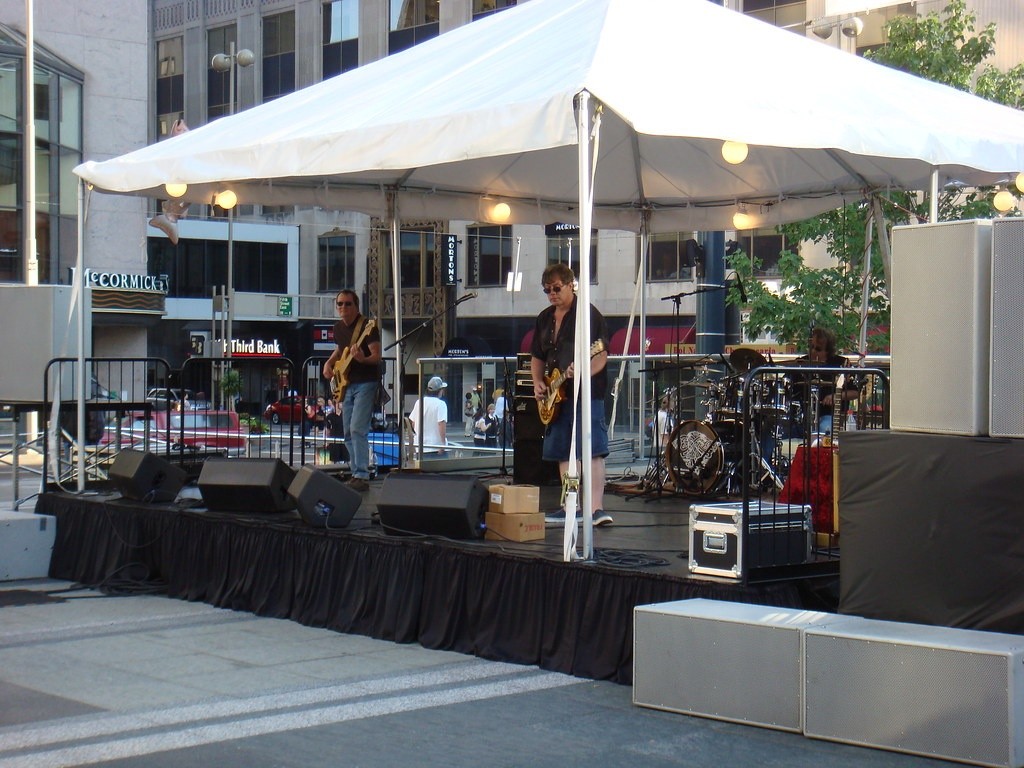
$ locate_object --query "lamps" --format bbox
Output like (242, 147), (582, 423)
(211, 190), (237, 209)
(993, 184), (1014, 211)
(166, 182), (187, 197)
(491, 203), (511, 221)
(722, 142), (748, 164)
(733, 204), (748, 230)
(1015, 173), (1024, 193)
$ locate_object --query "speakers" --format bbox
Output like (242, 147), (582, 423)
(685, 239), (701, 267)
(106, 447), (187, 505)
(512, 395), (563, 487)
(375, 473), (491, 540)
(287, 463), (363, 528)
(197, 457), (297, 514)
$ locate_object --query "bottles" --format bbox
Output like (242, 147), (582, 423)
(371, 452), (378, 477)
(812, 437), (823, 447)
(823, 431), (831, 447)
(843, 410), (857, 432)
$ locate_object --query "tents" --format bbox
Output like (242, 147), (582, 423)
(71, 0), (1024, 561)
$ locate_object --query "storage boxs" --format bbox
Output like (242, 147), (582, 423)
(488, 484), (539, 514)
(484, 513), (545, 542)
(688, 501), (813, 579)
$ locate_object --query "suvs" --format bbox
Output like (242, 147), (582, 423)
(99, 403), (247, 458)
(146, 387), (214, 410)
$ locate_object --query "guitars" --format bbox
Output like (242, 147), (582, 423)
(329, 319), (376, 403)
(537, 337), (609, 425)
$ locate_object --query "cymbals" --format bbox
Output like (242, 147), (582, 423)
(680, 380), (716, 388)
(773, 359), (831, 366)
(794, 379), (833, 386)
(730, 348), (768, 378)
(637, 359), (723, 372)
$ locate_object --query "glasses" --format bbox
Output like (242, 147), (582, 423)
(337, 301), (353, 306)
(543, 284), (564, 293)
(808, 344), (822, 352)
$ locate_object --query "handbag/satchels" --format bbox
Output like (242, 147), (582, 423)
(465, 407), (473, 414)
(474, 427), (485, 435)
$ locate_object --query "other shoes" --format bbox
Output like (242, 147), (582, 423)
(545, 508), (583, 522)
(344, 477), (369, 491)
(592, 509), (613, 525)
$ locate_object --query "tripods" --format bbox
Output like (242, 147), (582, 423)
(625, 283), (785, 504)
(478, 357), (516, 485)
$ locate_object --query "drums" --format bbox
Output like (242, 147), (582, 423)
(751, 377), (792, 415)
(712, 410), (751, 434)
(666, 420), (750, 495)
(717, 376), (756, 420)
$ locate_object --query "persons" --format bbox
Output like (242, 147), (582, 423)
(464, 389), (515, 458)
(323, 289), (383, 491)
(296, 396), (351, 465)
(527, 264), (614, 526)
(654, 397), (674, 464)
(790, 327), (867, 433)
(176, 392), (210, 412)
(409, 377), (449, 461)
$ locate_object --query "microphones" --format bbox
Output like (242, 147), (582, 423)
(683, 473), (693, 481)
(721, 353), (735, 374)
(769, 353), (776, 368)
(453, 291), (478, 305)
(737, 274), (747, 302)
(512, 398), (518, 415)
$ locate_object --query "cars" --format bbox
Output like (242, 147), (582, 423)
(266, 395), (328, 425)
(856, 393), (883, 427)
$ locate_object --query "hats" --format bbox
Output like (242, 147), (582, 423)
(428, 377), (447, 391)
(473, 388), (477, 390)
